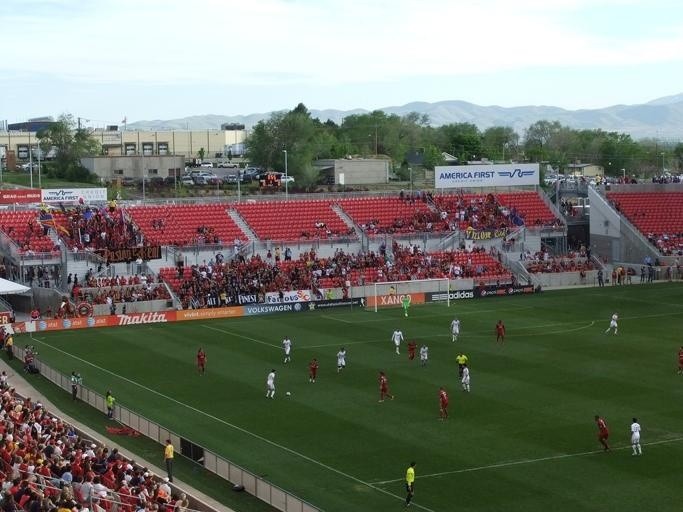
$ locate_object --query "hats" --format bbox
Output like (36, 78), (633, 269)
(57, 440), (63, 445)
(163, 477), (170, 483)
(81, 454), (88, 459)
(89, 443), (97, 449)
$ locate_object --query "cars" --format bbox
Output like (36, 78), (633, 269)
(119, 161), (296, 187)
(543, 175), (620, 186)
(3, 163), (41, 172)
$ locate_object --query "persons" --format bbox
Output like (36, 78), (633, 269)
(602, 309), (620, 337)
(401, 459), (423, 506)
(647, 231), (681, 257)
(174, 189), (524, 310)
(261, 332), (349, 400)
(2, 325), (204, 511)
(376, 316), (509, 418)
(598, 255), (681, 288)
(591, 412), (612, 453)
(627, 414), (646, 458)
(520, 200), (609, 274)
(611, 199), (621, 211)
(2, 197), (171, 323)
(596, 174), (682, 185)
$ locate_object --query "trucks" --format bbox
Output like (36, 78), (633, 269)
(0, 146), (8, 161)
(158, 146), (167, 155)
(18, 147), (59, 162)
(125, 146), (135, 156)
(223, 144), (247, 158)
(142, 145), (152, 156)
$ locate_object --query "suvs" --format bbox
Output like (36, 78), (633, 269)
(214, 152), (225, 158)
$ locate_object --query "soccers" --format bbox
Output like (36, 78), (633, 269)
(286, 392), (290, 396)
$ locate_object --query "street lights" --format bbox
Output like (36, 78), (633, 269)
(77, 116), (90, 140)
(660, 152), (667, 183)
(281, 144), (288, 201)
(621, 168), (626, 185)
(654, 138), (665, 181)
(408, 166), (413, 192)
(36, 139), (44, 188)
